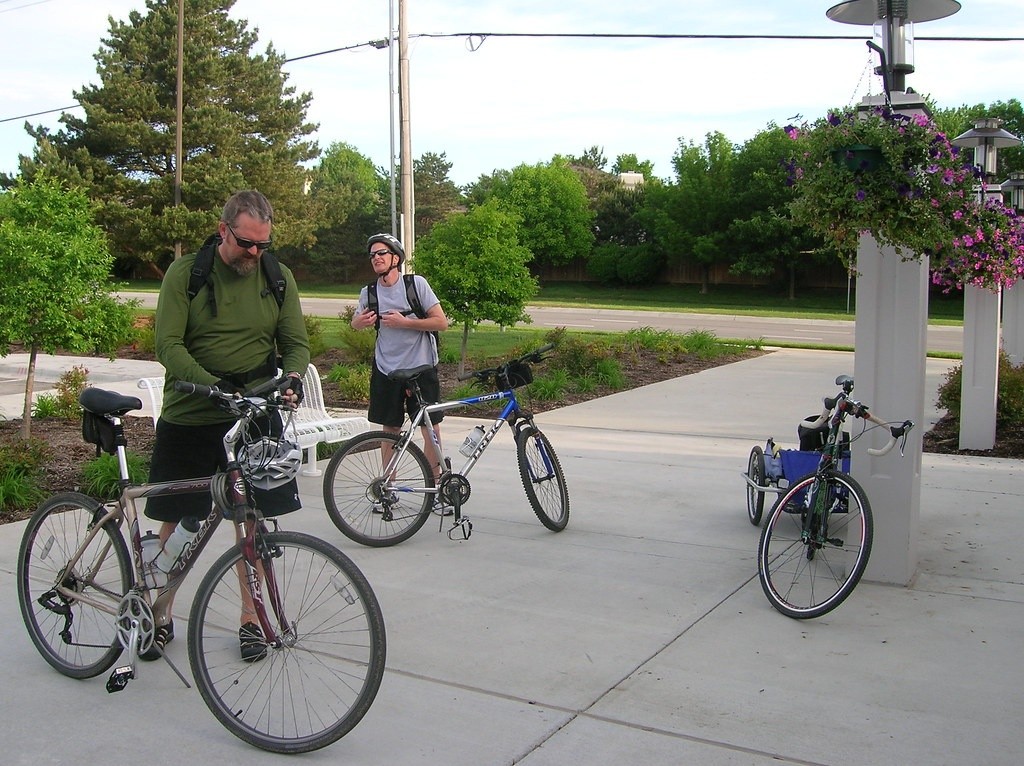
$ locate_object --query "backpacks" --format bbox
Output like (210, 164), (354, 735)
(798, 415), (842, 451)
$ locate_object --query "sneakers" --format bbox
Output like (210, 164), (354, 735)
(135, 619), (174, 661)
(431, 496), (454, 516)
(371, 491), (399, 513)
(239, 622), (267, 663)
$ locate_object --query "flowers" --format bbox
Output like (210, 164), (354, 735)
(932, 182), (1024, 295)
(774, 112), (984, 278)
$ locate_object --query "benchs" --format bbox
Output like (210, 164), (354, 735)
(137, 364), (370, 477)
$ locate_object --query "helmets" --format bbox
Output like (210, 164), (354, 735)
(366, 233), (404, 266)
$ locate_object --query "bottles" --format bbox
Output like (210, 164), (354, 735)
(459, 425), (485, 457)
(157, 515), (201, 573)
(140, 530), (168, 588)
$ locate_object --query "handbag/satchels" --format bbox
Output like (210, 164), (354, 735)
(764, 438), (784, 482)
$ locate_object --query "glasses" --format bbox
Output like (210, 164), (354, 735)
(368, 249), (395, 260)
(226, 223), (273, 250)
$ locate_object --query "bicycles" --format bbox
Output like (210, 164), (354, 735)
(742, 374), (917, 619)
(323, 341), (570, 546)
(17, 379), (388, 755)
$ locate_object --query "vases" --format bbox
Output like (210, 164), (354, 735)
(836, 143), (876, 173)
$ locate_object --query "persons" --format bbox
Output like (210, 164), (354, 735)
(350, 234), (458, 517)
(138, 190), (310, 661)
